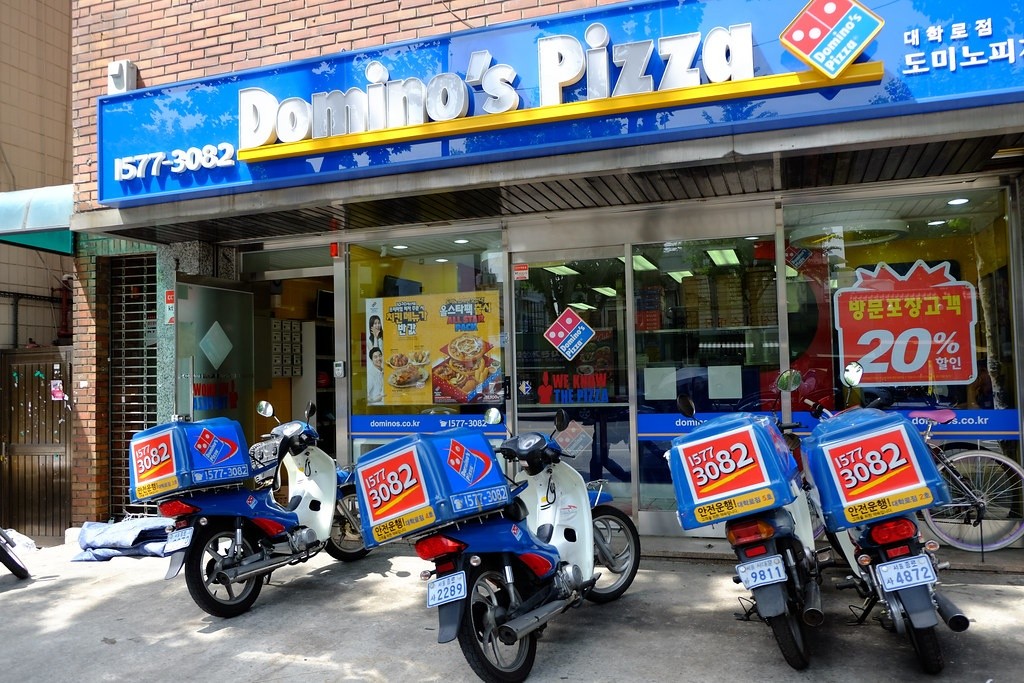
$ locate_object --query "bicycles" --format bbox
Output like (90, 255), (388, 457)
(802, 396), (1024, 562)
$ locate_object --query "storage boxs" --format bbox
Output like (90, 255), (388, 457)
(354, 428), (513, 549)
(669, 412), (804, 530)
(129, 416), (253, 504)
(801, 407), (951, 533)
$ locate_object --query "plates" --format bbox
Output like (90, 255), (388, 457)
(576, 343), (610, 375)
(387, 369), (428, 387)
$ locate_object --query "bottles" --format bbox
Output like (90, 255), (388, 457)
(698, 341), (778, 356)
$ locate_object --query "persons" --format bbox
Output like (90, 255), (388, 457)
(366, 347), (383, 403)
(367, 315), (382, 353)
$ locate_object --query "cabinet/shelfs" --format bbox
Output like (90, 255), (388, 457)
(291, 321), (336, 459)
(515, 325), (778, 397)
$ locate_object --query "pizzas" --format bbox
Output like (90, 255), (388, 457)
(448, 335), (486, 362)
(449, 357), (485, 377)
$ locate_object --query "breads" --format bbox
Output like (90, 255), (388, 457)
(460, 367), (489, 392)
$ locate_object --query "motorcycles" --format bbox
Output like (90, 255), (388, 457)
(664, 388), (829, 670)
(352, 406), (641, 683)
(129, 400), (367, 617)
(779, 364), (969, 644)
